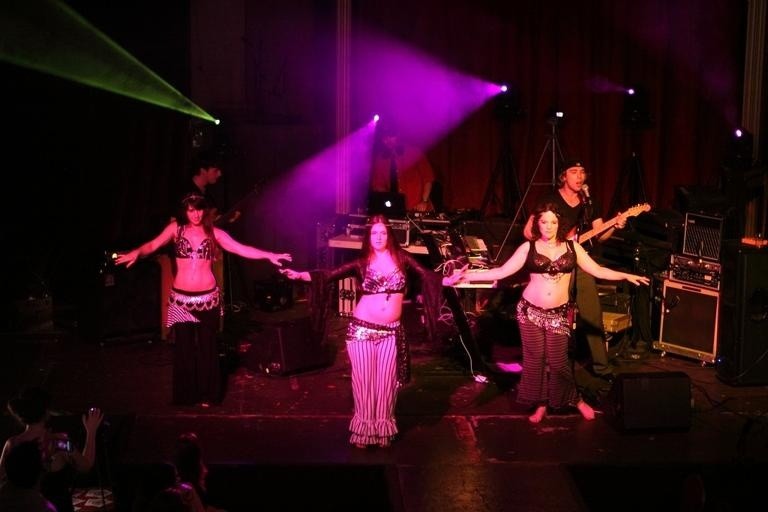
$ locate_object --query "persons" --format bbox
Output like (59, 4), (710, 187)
(151, 153), (243, 341)
(114, 190), (293, 409)
(453, 200), (650, 423)
(0, 383), (229, 512)
(369, 125), (435, 212)
(522, 156), (629, 383)
(277, 214), (470, 450)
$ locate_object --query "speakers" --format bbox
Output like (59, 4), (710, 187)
(716, 238), (768, 387)
(242, 314), (336, 377)
(659, 279), (719, 366)
(602, 372), (692, 434)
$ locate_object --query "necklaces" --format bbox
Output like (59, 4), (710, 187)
(538, 238), (558, 249)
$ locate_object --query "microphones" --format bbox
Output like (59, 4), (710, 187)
(666, 297), (679, 313)
(581, 182), (591, 203)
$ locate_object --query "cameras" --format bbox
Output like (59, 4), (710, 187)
(53, 438), (70, 452)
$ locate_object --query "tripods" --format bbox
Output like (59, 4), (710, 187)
(495, 124), (567, 263)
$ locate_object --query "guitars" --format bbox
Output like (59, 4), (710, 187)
(567, 201), (651, 254)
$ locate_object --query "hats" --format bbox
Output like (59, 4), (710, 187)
(563, 159), (586, 170)
